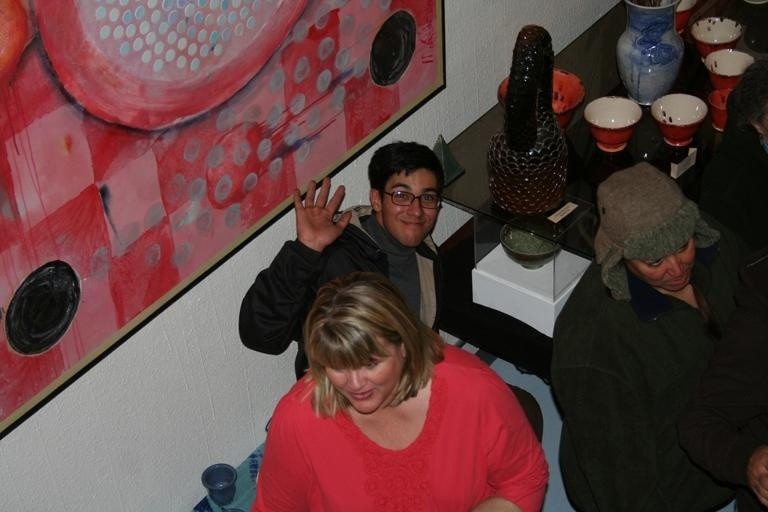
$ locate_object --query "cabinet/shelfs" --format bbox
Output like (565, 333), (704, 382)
(434, 0), (767, 385)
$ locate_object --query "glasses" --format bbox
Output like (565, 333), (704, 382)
(381, 190), (442, 209)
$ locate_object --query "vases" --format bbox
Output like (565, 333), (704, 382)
(618, 1), (684, 106)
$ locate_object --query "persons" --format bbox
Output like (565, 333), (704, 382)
(551, 162), (767, 512)
(674, 245), (767, 511)
(237, 139), (546, 446)
(695, 56), (768, 255)
(252, 272), (550, 511)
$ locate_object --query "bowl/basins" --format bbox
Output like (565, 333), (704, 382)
(496, 65), (586, 134)
(499, 218), (562, 271)
(675, 0), (696, 34)
(704, 48), (755, 85)
(583, 94), (642, 152)
(690, 16), (743, 62)
(648, 93), (708, 147)
(708, 87), (735, 132)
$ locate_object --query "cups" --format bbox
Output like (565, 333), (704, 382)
(200, 463), (236, 505)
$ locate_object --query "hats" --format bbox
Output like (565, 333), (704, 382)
(595, 161), (720, 301)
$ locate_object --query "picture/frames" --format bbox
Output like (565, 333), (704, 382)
(0, 0), (448, 439)
